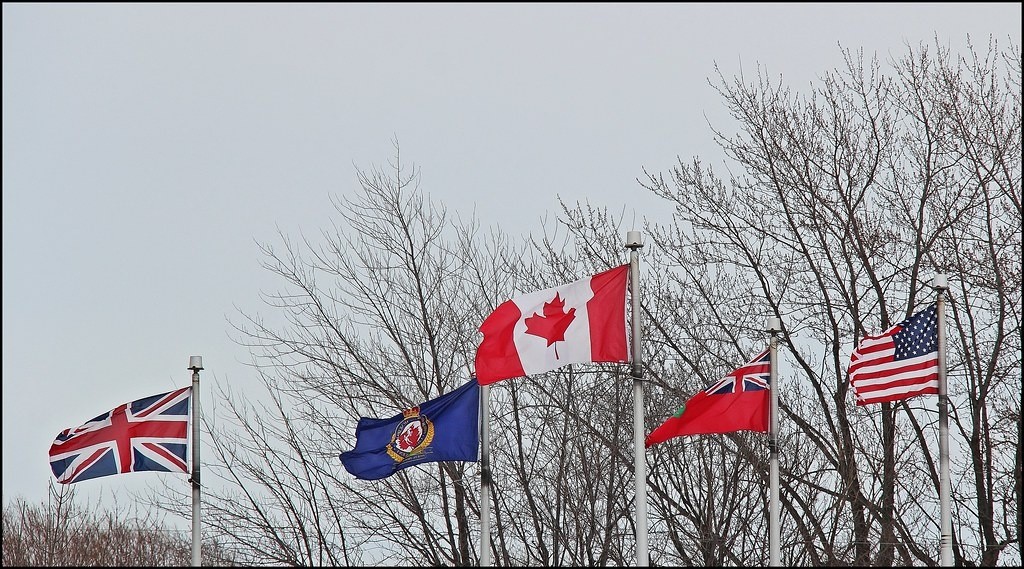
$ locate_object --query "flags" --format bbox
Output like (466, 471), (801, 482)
(644, 346), (772, 446)
(847, 304), (942, 407)
(47, 385), (194, 488)
(476, 263), (634, 384)
(340, 375), (485, 480)
